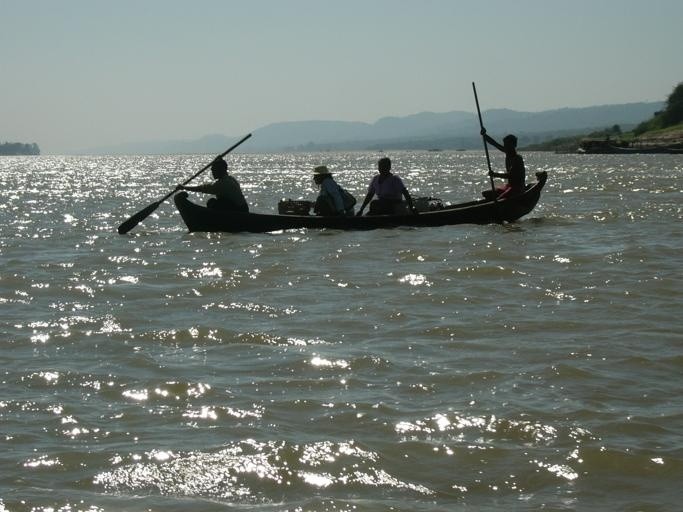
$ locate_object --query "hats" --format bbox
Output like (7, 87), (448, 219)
(309, 165), (334, 175)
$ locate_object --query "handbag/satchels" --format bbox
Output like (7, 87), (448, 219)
(313, 189), (356, 210)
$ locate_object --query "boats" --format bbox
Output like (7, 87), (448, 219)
(173, 166), (550, 233)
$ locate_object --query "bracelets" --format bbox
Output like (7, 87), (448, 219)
(360, 206), (364, 210)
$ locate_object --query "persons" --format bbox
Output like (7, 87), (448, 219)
(354, 158), (419, 218)
(173, 159), (249, 211)
(307, 166), (355, 217)
(479, 126), (525, 200)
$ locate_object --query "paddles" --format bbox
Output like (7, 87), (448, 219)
(117, 133), (253, 234)
(472, 81), (505, 225)
